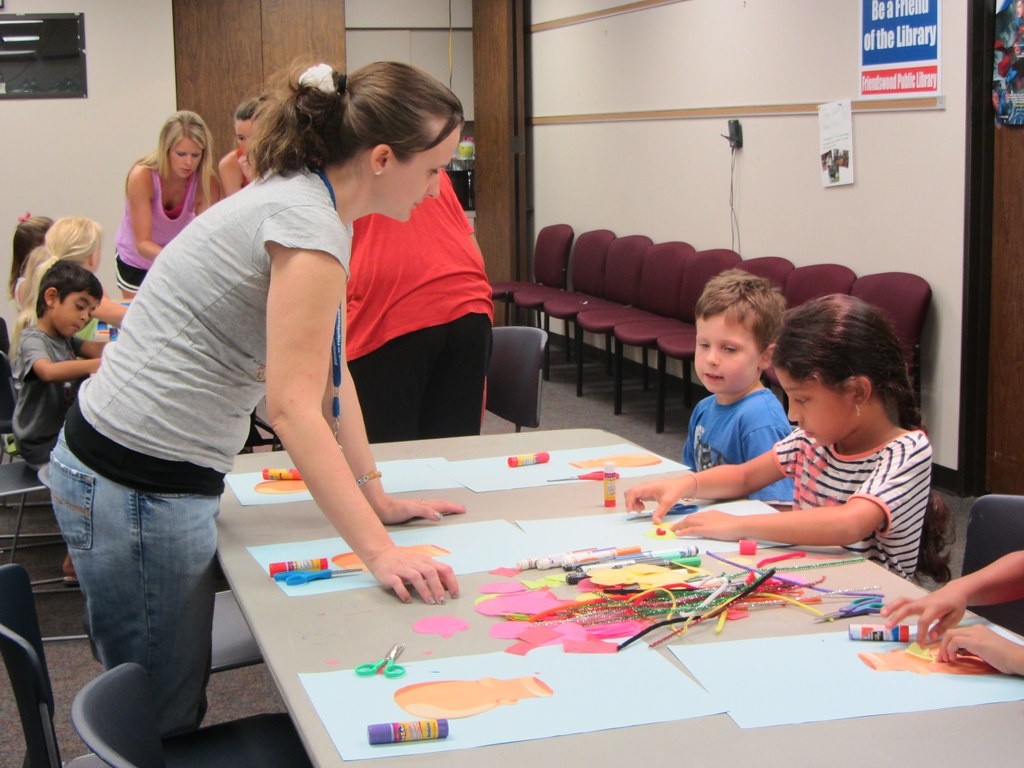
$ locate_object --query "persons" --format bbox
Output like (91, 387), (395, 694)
(10, 95), (495, 587)
(680, 269), (795, 512)
(882, 549), (1024, 679)
(625, 294), (952, 582)
(46, 60), (464, 741)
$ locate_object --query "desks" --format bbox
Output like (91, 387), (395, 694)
(215, 429), (1024, 768)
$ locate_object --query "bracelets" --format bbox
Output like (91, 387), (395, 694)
(680, 474), (699, 502)
(357, 470), (383, 486)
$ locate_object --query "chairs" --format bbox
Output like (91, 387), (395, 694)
(485, 224), (933, 433)
(960, 493), (1024, 638)
(0, 317), (313, 768)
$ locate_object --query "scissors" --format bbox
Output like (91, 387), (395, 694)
(545, 470), (620, 484)
(353, 643), (407, 679)
(273, 568), (364, 585)
(814, 596), (884, 627)
(627, 503), (698, 522)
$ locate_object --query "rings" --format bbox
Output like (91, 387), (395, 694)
(418, 497), (423, 500)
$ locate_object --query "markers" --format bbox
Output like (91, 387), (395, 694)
(563, 546), (702, 582)
(517, 543), (641, 568)
